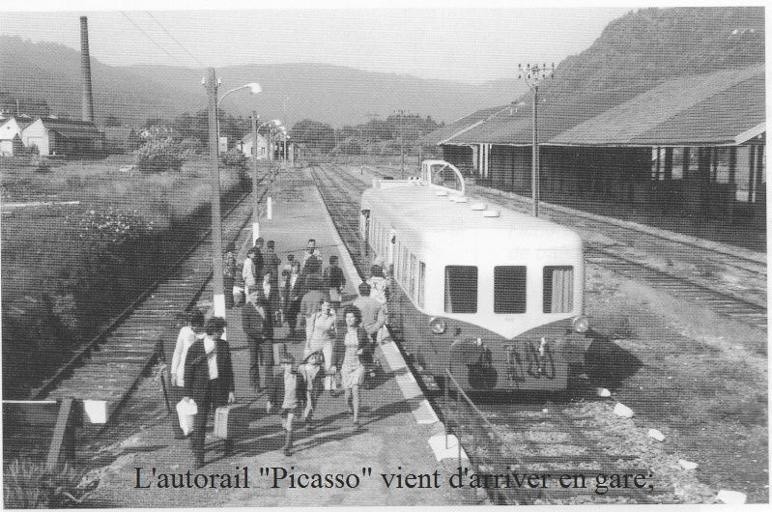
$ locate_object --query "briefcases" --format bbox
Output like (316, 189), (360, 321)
(214, 402), (250, 441)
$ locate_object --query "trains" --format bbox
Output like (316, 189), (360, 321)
(357, 157), (594, 394)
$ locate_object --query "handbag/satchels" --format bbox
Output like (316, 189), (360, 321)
(176, 398), (198, 437)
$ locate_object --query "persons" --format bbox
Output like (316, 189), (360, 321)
(167, 236), (392, 470)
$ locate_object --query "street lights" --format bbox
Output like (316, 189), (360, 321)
(203, 65), (263, 341)
(249, 109), (291, 251)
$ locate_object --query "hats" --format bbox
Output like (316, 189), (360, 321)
(280, 353), (295, 364)
